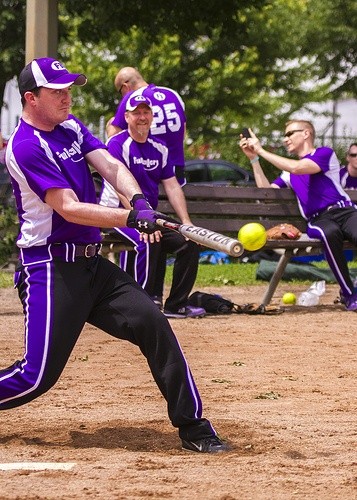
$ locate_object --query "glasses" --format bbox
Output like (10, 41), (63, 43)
(349, 153), (357, 157)
(284, 130), (304, 138)
(118, 79), (130, 95)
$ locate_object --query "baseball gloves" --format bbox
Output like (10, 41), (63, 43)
(265, 223), (303, 240)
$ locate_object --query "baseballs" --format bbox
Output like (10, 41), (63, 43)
(239, 223), (266, 252)
(283, 293), (296, 305)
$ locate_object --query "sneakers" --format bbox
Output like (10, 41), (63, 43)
(345, 297), (357, 311)
(182, 435), (231, 454)
(162, 304), (207, 319)
(151, 296), (164, 309)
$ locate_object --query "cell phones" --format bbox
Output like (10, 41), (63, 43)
(242, 128), (253, 144)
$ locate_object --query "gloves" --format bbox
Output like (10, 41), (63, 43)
(126, 209), (171, 235)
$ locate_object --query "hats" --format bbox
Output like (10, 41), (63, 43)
(18, 57), (87, 96)
(125, 95), (154, 112)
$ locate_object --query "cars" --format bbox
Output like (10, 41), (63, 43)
(181, 159), (258, 187)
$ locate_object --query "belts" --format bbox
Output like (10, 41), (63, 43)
(50, 244), (98, 259)
(322, 201), (351, 212)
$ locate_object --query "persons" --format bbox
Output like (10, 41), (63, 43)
(239, 120), (357, 311)
(97, 95), (206, 318)
(339, 143), (357, 190)
(0, 57), (232, 452)
(106, 66), (186, 185)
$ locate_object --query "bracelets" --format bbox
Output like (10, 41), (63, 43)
(251, 156), (259, 164)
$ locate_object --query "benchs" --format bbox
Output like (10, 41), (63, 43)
(92, 179), (357, 308)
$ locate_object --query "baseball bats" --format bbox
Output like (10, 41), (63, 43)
(157, 218), (245, 257)
